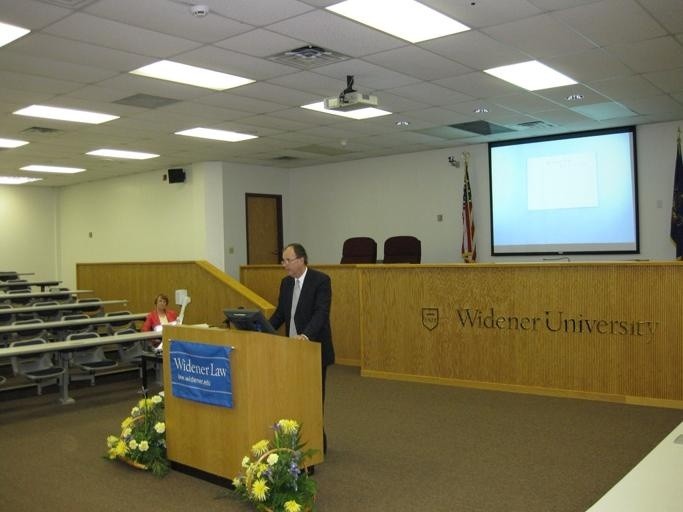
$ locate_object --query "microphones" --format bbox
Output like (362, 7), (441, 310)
(223, 306), (244, 323)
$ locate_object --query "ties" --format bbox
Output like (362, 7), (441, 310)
(289, 280), (301, 338)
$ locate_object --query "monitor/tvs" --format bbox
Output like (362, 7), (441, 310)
(223, 309), (278, 335)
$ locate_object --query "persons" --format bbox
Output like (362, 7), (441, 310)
(262, 244), (336, 456)
(142, 293), (177, 350)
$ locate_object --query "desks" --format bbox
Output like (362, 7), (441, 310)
(0, 280), (63, 291)
(0, 272), (35, 279)
(0, 313), (151, 335)
(0, 289), (94, 302)
(1, 299), (128, 315)
(0, 331), (162, 405)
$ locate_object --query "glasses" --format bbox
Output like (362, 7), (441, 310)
(281, 258), (305, 265)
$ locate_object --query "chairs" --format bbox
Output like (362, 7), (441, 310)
(66, 333), (116, 387)
(6, 280), (31, 293)
(61, 315), (92, 336)
(383, 235), (421, 265)
(105, 311), (134, 336)
(115, 330), (146, 384)
(340, 237), (378, 264)
(48, 288), (73, 304)
(10, 339), (65, 397)
(11, 319), (45, 339)
(0, 305), (16, 326)
(33, 302), (62, 321)
(76, 299), (102, 318)
(7, 289), (32, 307)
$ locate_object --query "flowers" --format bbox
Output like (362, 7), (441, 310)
(101, 391), (171, 481)
(222, 417), (317, 511)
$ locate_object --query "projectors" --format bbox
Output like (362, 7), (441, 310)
(324, 76), (378, 112)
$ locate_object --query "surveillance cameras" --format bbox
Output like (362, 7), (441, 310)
(448, 156), (456, 165)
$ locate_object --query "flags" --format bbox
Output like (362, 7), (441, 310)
(460, 160), (477, 262)
(670, 137), (683, 260)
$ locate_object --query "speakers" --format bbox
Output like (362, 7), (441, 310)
(167, 168), (185, 183)
